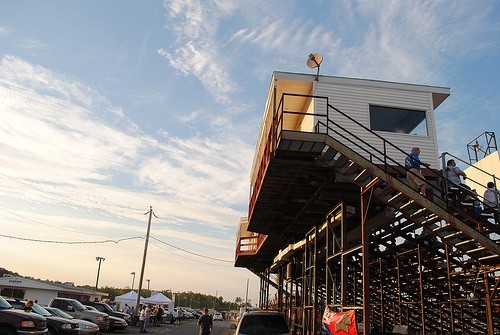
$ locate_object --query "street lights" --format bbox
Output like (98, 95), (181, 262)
(95, 256), (106, 291)
(130, 271), (136, 291)
(146, 279), (150, 291)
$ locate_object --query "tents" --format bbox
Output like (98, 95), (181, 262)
(115, 290), (145, 308)
(144, 293), (174, 313)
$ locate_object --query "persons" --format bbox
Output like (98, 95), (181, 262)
(14, 298), (19, 305)
(467, 189), (480, 207)
(172, 308), (182, 326)
(107, 301), (117, 311)
(152, 305), (164, 326)
(22, 300), (33, 310)
(483, 181), (500, 224)
(34, 300), (38, 303)
(447, 159), (471, 201)
(405, 147), (430, 196)
(122, 304), (135, 315)
(197, 307), (213, 335)
(227, 313), (237, 321)
(139, 303), (153, 333)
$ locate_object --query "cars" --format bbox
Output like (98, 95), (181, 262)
(5, 296), (131, 335)
(164, 307), (223, 322)
(229, 310), (292, 335)
(0, 295), (49, 335)
